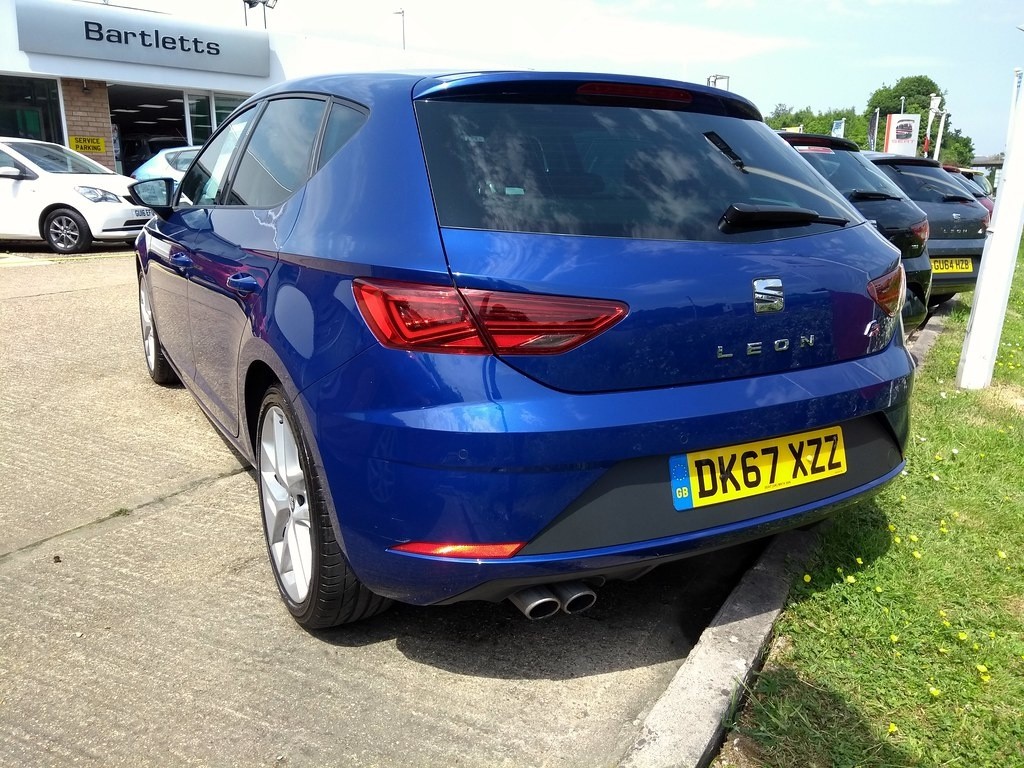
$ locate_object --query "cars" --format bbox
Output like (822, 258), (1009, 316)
(0, 137), (159, 254)
(130, 145), (203, 192)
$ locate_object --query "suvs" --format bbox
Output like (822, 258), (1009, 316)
(941, 164), (995, 221)
(775, 130), (933, 341)
(859, 149), (992, 306)
(127, 69), (916, 633)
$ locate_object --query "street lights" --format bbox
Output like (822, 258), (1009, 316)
(392, 6), (407, 52)
